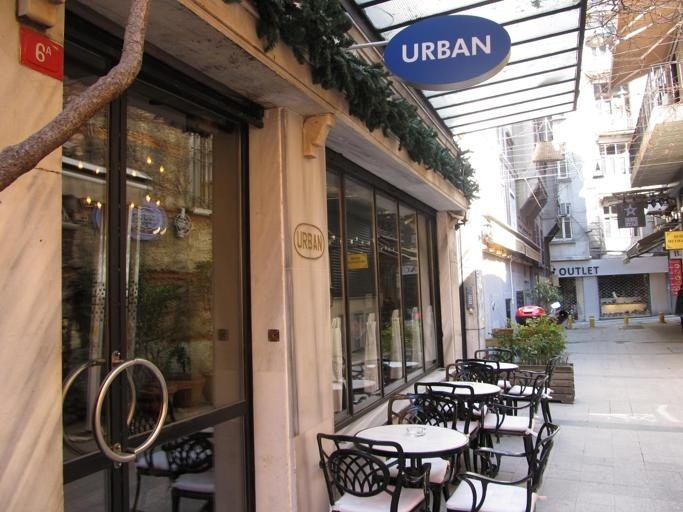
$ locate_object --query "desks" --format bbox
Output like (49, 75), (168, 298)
(354, 423), (471, 511)
(342, 378), (375, 393)
(462, 358), (520, 373)
(383, 360), (418, 368)
(416, 378), (502, 417)
(132, 448), (213, 512)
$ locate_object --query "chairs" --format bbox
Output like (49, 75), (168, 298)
(486, 375), (550, 434)
(474, 346), (514, 387)
(444, 422), (560, 512)
(444, 360), (495, 385)
(164, 432), (217, 512)
(386, 392), (458, 483)
(415, 381), (483, 473)
(316, 432), (433, 512)
